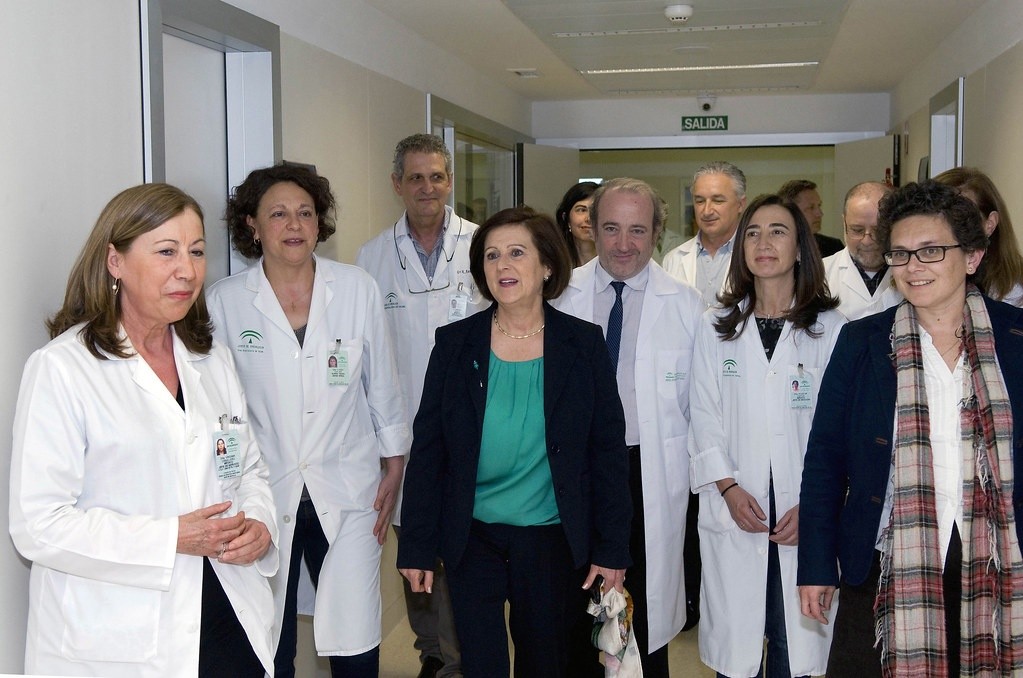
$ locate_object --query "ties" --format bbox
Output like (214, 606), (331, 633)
(606, 281), (627, 380)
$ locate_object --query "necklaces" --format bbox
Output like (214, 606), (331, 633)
(494, 306), (545, 339)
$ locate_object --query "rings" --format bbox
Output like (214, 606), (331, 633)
(219, 543), (226, 558)
(739, 522), (744, 526)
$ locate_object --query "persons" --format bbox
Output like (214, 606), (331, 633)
(452, 300), (456, 308)
(204, 161), (411, 678)
(356, 133), (493, 678)
(216, 439), (228, 456)
(796, 177), (1023, 678)
(928, 165), (1023, 309)
(9, 183), (279, 678)
(546, 160), (906, 678)
(396, 205), (633, 678)
(328, 356), (337, 368)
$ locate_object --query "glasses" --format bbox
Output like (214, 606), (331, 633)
(844, 219), (878, 241)
(404, 245), (450, 293)
(881, 245), (962, 266)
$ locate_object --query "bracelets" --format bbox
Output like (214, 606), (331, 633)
(720, 483), (739, 497)
